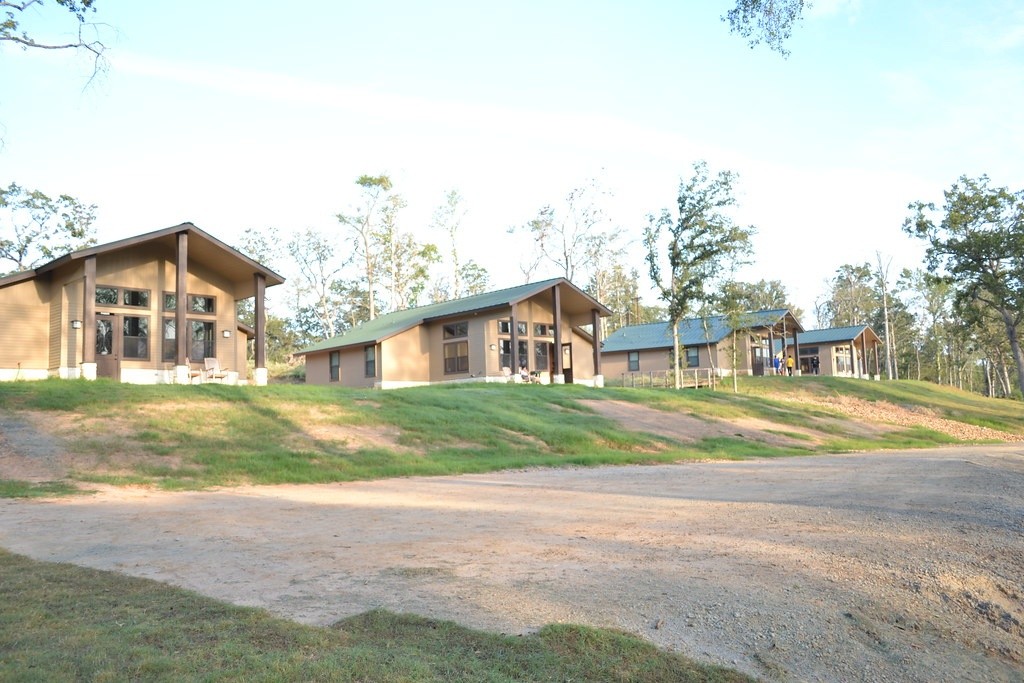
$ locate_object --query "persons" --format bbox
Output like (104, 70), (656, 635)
(786, 355), (794, 375)
(780, 355), (786, 376)
(774, 355), (780, 375)
(520, 366), (543, 384)
(811, 356), (820, 374)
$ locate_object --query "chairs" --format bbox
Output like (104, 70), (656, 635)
(519, 367), (529, 384)
(502, 366), (511, 380)
(186, 357), (203, 385)
(204, 357), (229, 383)
